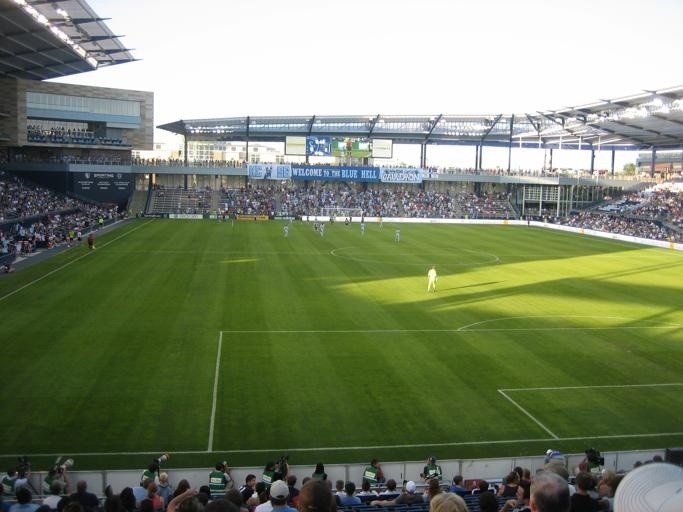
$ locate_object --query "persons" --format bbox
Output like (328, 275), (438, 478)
(427, 264), (438, 293)
(1, 150), (682, 272)
(2, 449), (682, 511)
(26, 123), (84, 137)
(346, 139), (352, 156)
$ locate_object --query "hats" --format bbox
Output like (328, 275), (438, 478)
(406, 481), (416, 492)
(613, 462), (683, 512)
(270, 480), (289, 500)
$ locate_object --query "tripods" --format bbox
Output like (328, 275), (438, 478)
(17, 471), (39, 494)
